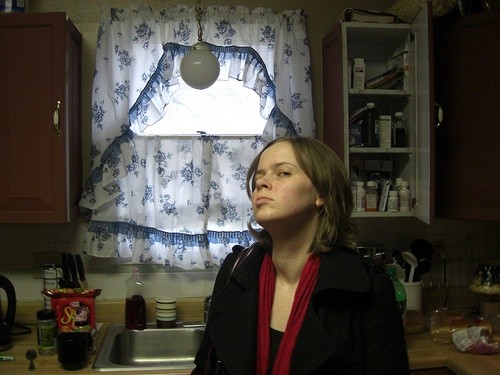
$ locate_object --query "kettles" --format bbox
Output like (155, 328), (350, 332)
(0, 273), (17, 351)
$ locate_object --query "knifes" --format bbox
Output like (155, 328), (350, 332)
(58, 251), (85, 289)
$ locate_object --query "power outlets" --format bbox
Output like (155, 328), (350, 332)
(431, 240), (448, 259)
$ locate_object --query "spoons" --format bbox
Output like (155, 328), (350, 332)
(26, 349), (37, 371)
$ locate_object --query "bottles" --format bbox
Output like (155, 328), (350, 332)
(124, 267), (146, 330)
(392, 112), (406, 148)
(385, 264), (407, 320)
(361, 103), (379, 147)
(351, 177), (410, 213)
(36, 309), (57, 356)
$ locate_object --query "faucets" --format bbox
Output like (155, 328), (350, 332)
(180, 295), (212, 328)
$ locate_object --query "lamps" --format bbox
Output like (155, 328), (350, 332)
(180, 0), (220, 89)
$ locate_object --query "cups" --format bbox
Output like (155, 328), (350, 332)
(155, 296), (177, 328)
(56, 332), (87, 370)
(402, 280), (423, 312)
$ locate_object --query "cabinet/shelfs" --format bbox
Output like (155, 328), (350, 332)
(322, 0), (443, 225)
(436, 9), (500, 221)
(0, 11), (83, 224)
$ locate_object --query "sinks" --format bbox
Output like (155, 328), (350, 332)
(91, 323), (205, 372)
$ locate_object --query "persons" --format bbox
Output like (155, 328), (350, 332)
(190, 135), (410, 375)
